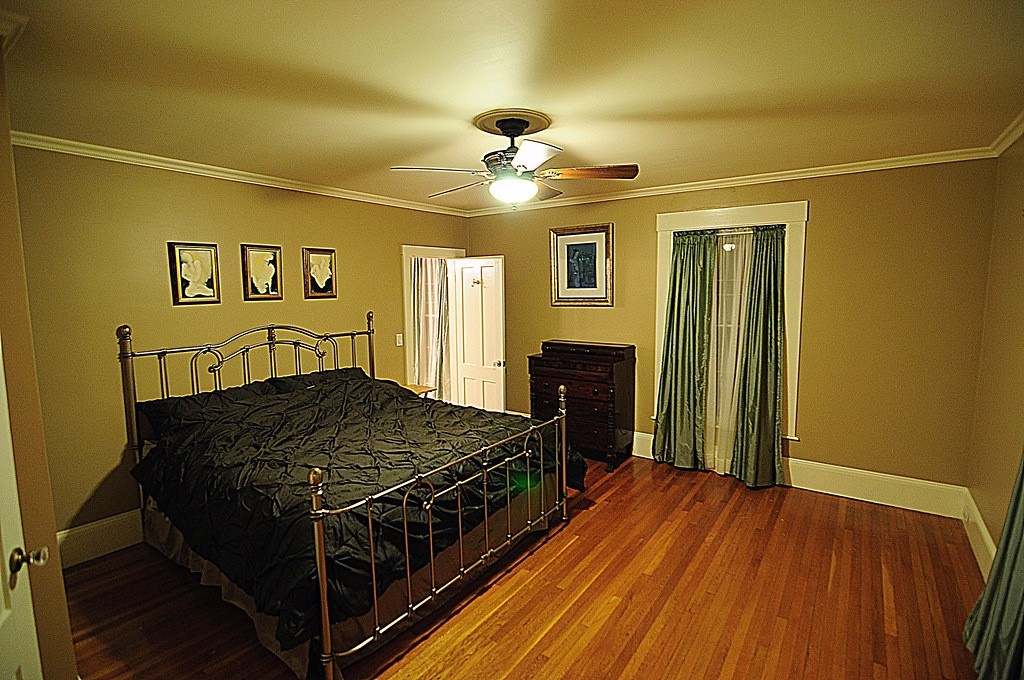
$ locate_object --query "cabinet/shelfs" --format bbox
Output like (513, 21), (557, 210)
(527, 338), (637, 474)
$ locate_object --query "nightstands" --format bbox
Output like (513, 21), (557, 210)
(406, 384), (437, 399)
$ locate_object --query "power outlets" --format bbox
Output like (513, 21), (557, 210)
(396, 333), (403, 347)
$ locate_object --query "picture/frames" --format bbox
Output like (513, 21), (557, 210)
(548, 222), (615, 308)
(167, 241), (221, 306)
(240, 243), (284, 302)
(301, 247), (337, 300)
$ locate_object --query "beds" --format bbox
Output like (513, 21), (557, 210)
(117, 310), (588, 680)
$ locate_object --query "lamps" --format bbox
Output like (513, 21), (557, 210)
(489, 172), (539, 212)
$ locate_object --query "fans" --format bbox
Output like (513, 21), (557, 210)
(389, 108), (640, 202)
